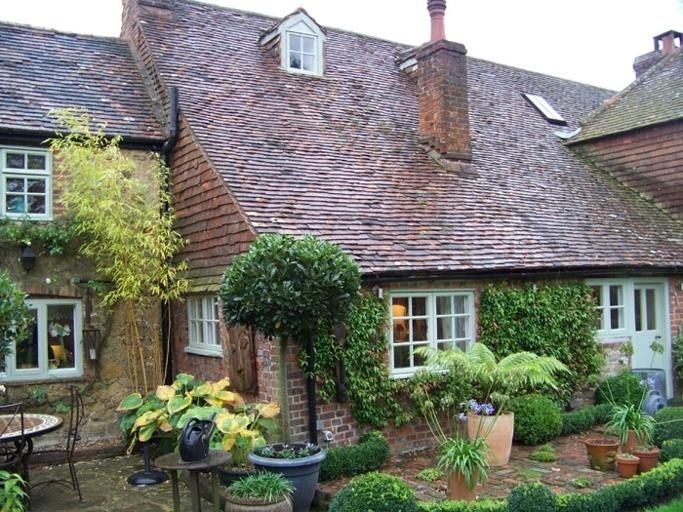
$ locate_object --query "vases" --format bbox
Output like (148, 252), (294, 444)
(585, 438), (621, 471)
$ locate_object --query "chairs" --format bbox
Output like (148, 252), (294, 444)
(21, 385), (84, 510)
(0, 400), (26, 510)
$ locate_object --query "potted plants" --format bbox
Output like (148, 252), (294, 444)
(216, 232), (363, 512)
(587, 341), (665, 455)
(411, 342), (573, 467)
(415, 383), (508, 503)
(615, 453), (640, 478)
(222, 467), (295, 512)
(633, 443), (661, 474)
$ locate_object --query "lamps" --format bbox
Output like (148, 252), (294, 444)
(20, 241), (36, 274)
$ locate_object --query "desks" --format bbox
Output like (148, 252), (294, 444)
(154, 449), (233, 512)
(0, 413), (64, 480)
